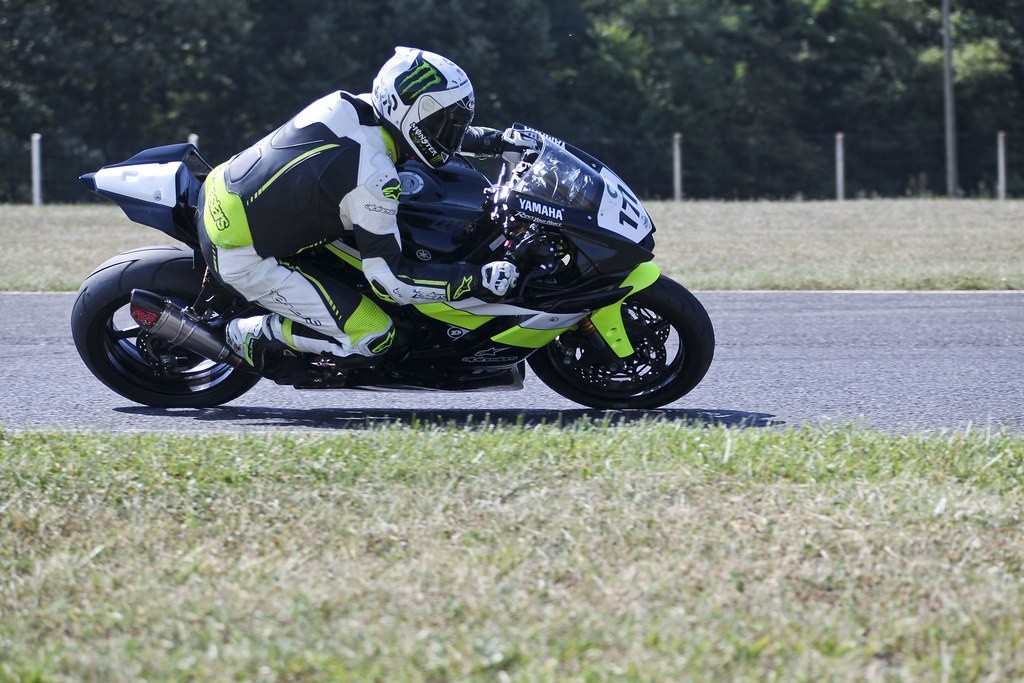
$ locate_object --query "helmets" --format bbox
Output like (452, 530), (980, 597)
(371, 46), (474, 172)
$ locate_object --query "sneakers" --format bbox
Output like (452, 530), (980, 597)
(224, 314), (281, 378)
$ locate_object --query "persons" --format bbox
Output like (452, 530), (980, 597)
(193, 45), (522, 376)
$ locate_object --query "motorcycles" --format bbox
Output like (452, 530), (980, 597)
(71, 122), (716, 410)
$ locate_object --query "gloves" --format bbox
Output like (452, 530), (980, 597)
(475, 261), (520, 303)
(501, 127), (540, 166)
